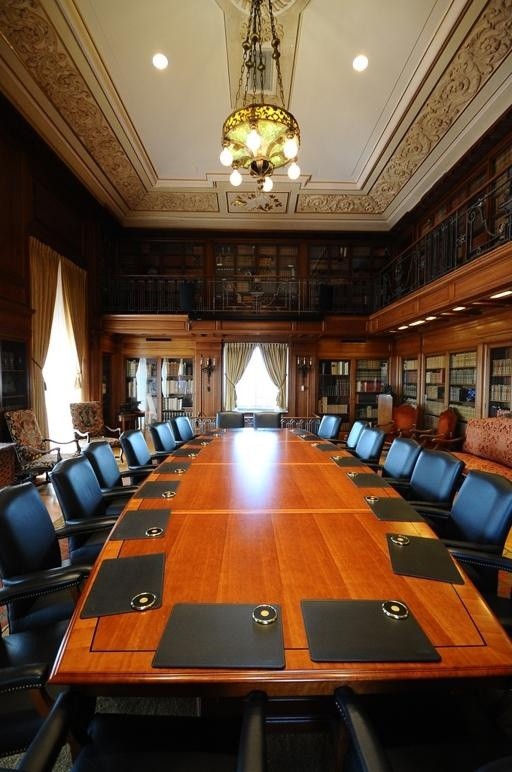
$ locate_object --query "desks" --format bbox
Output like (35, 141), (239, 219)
(44, 424), (511, 681)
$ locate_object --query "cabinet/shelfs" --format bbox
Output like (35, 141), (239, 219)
(421, 349), (448, 424)
(112, 240), (395, 314)
(124, 350), (198, 423)
(395, 135), (512, 298)
(317, 357), (396, 423)
(400, 353), (421, 406)
(448, 346), (482, 433)
(484, 341), (512, 418)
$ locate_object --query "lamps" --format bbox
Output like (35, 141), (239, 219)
(296, 354), (313, 368)
(215, 2), (306, 194)
(198, 355), (216, 371)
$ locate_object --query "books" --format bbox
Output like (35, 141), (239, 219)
(316, 346), (511, 435)
(123, 354), (194, 432)
(114, 234), (386, 318)
(383, 144), (511, 304)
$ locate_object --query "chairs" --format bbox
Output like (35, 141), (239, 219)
(0, 400), (512, 772)
